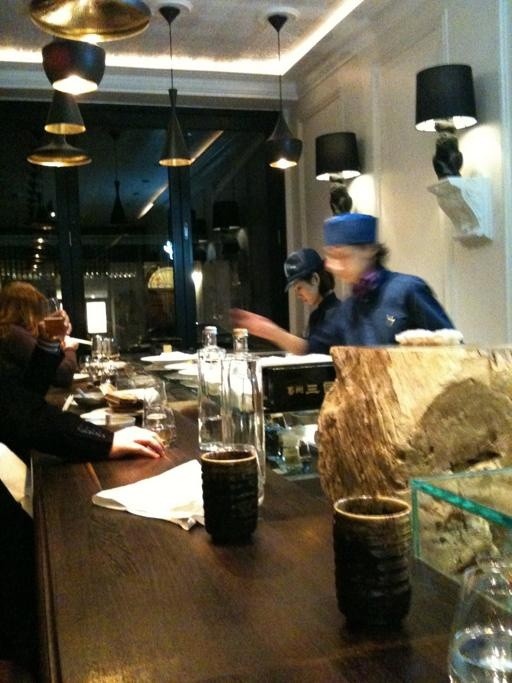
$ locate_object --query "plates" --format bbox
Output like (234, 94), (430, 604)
(71, 372), (90, 382)
(138, 347), (199, 377)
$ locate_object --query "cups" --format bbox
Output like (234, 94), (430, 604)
(199, 449), (260, 548)
(446, 561), (512, 682)
(78, 354), (180, 455)
(329, 494), (414, 630)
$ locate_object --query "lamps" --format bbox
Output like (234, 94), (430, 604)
(26, 2), (152, 168)
(315, 133), (361, 215)
(414, 66), (479, 180)
(157, 5), (192, 168)
(264, 14), (304, 168)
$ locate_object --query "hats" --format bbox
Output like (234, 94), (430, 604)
(281, 245), (325, 290)
(321, 212), (377, 246)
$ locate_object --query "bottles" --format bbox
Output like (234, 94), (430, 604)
(219, 327), (267, 506)
(194, 325), (234, 454)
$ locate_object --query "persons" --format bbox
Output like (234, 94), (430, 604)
(47, 334), (81, 389)
(229, 213), (462, 356)
(286, 248), (342, 336)
(0, 281), (166, 663)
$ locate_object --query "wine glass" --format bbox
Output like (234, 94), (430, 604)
(41, 297), (74, 352)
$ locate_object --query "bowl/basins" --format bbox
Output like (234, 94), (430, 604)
(73, 392), (106, 411)
